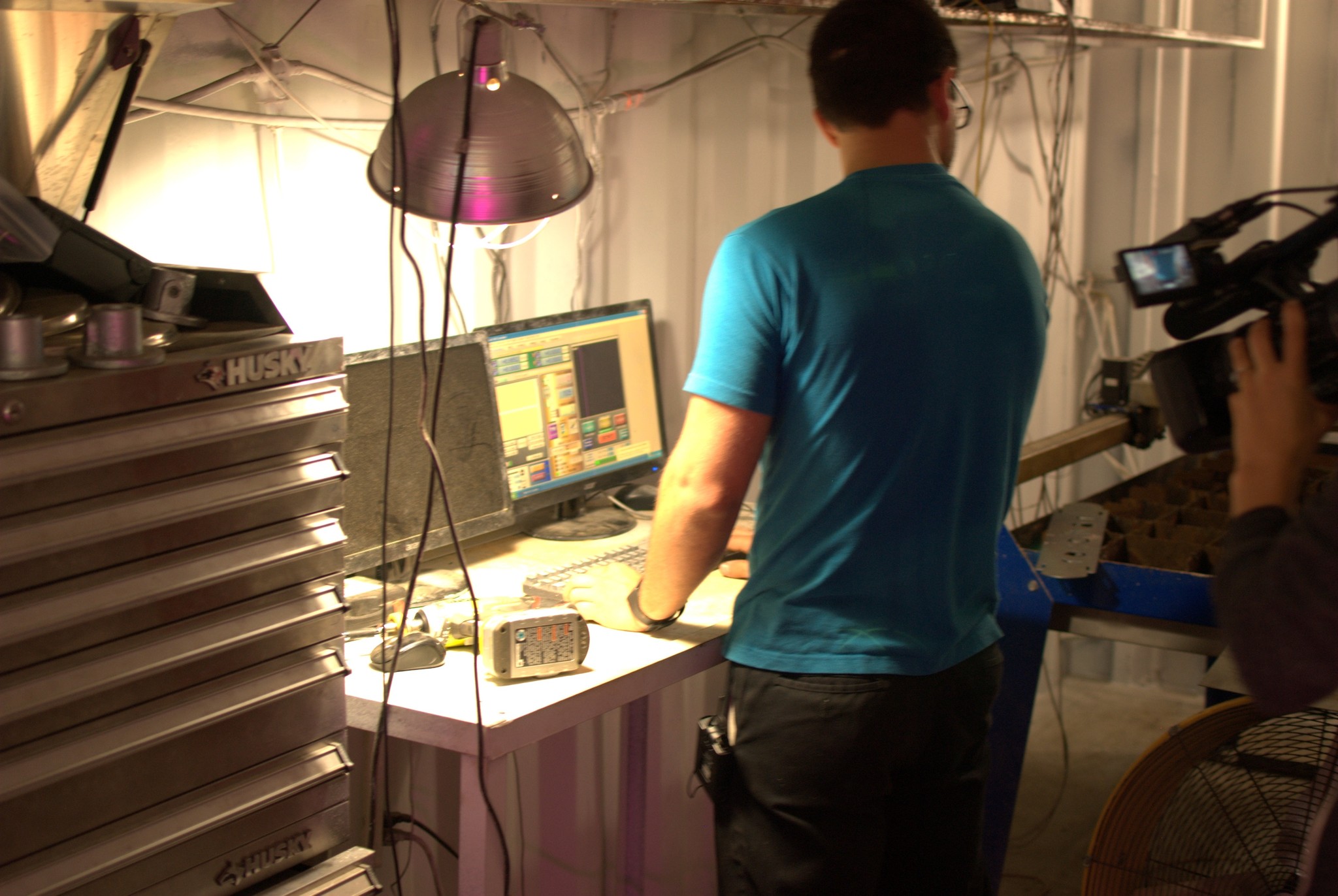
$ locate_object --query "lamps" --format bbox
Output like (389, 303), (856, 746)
(367, 16), (594, 250)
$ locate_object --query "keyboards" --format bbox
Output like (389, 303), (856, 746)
(519, 539), (744, 604)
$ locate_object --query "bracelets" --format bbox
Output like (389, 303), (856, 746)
(625, 580), (685, 632)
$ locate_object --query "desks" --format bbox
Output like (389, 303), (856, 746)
(345, 521), (745, 896)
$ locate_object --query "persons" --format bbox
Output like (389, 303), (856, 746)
(1220, 295), (1338, 709)
(564, 5), (1051, 896)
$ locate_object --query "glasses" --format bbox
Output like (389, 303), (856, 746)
(929, 72), (975, 131)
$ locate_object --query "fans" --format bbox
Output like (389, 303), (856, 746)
(1080, 697), (1338, 896)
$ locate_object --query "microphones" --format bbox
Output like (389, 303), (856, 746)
(1163, 202), (1337, 340)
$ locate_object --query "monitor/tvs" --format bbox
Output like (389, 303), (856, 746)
(333, 328), (513, 616)
(469, 299), (671, 542)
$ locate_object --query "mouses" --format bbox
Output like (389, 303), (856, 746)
(369, 631), (447, 673)
